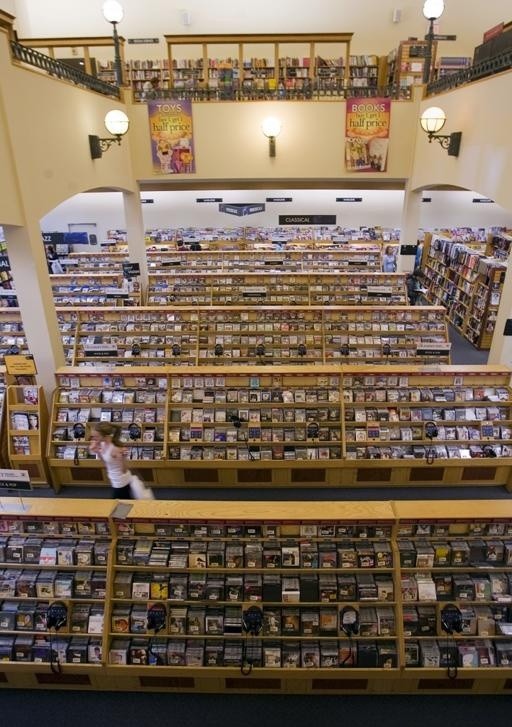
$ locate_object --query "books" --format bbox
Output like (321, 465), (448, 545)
(0, 225), (512, 668)
(100, 46), (474, 100)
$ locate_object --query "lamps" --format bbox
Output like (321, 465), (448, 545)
(86, 108), (132, 160)
(418, 103), (463, 158)
(259, 111), (284, 158)
(422, 0), (446, 84)
(98, 0), (129, 87)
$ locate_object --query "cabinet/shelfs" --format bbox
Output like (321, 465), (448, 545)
(42, 270), (411, 308)
(6, 384), (50, 488)
(417, 221), (512, 349)
(104, 56), (474, 101)
(0, 494), (512, 699)
(2, 304), (454, 366)
(63, 224), (403, 274)
(44, 363), (512, 493)
